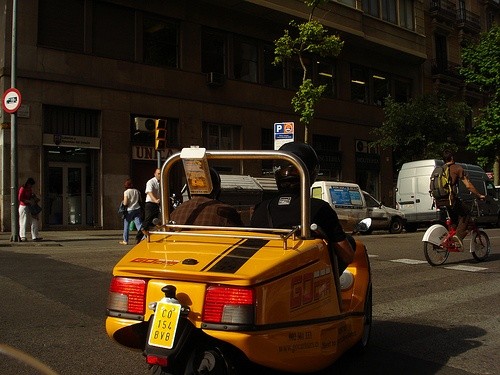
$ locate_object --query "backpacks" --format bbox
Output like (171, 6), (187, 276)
(430, 164), (459, 200)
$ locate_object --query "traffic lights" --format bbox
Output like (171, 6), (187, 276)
(155, 117), (168, 150)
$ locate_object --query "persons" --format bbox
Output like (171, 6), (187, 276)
(250, 142), (357, 276)
(134, 168), (161, 244)
(18, 178), (42, 242)
(433, 148), (487, 252)
(169, 165), (245, 227)
(118, 178), (146, 245)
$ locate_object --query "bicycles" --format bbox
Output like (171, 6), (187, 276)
(422, 197), (491, 268)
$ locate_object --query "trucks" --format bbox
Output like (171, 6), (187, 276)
(311, 180), (407, 232)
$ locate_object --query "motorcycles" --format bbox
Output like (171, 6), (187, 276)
(104, 147), (375, 375)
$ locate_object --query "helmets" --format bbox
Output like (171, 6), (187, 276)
(273, 142), (320, 186)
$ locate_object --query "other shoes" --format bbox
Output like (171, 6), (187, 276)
(120, 241), (127, 245)
(452, 235), (464, 252)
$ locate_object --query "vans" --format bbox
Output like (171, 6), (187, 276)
(396, 159), (500, 231)
